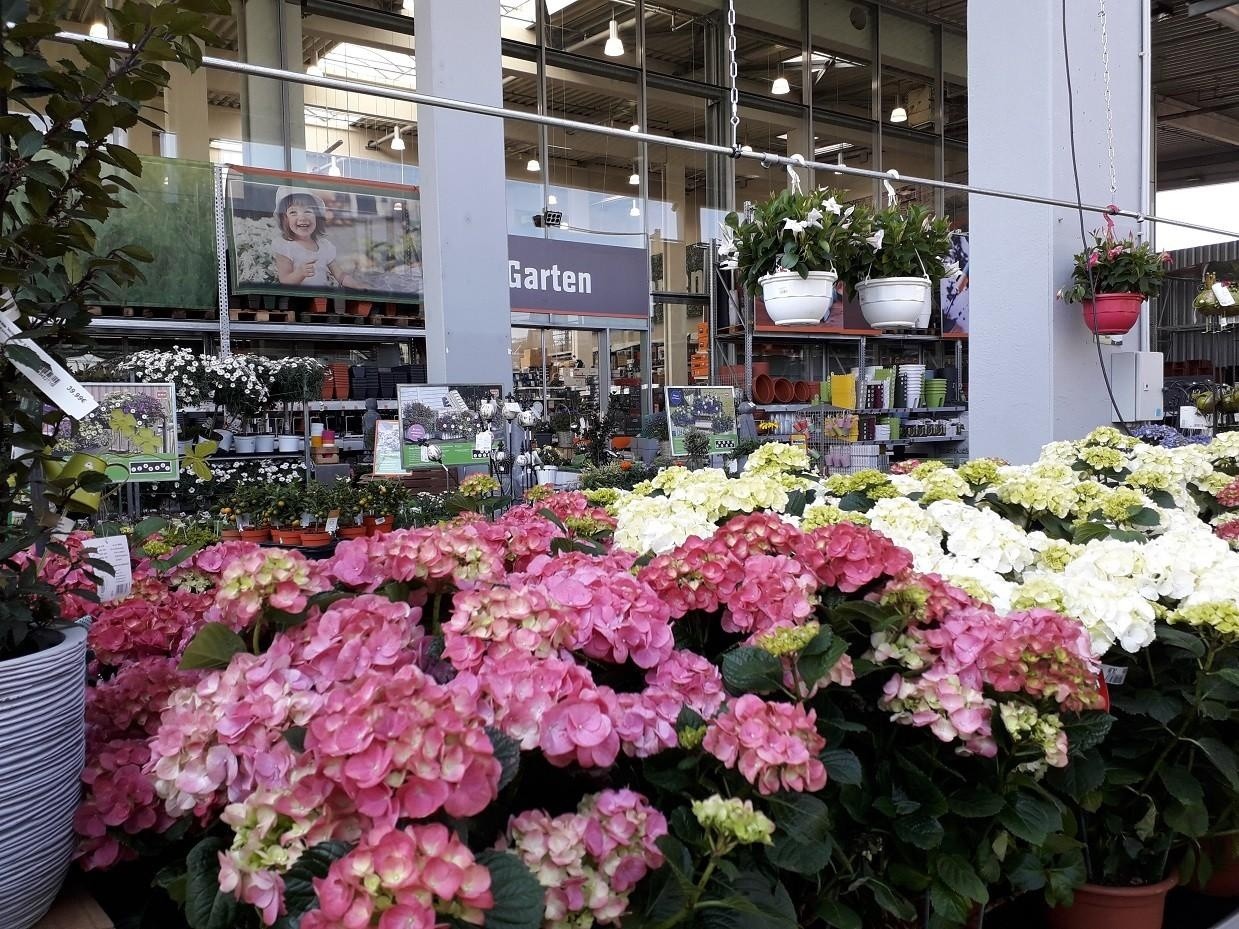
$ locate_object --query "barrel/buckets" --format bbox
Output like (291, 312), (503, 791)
(752, 374), (774, 404)
(720, 365), (745, 388)
(808, 384), (820, 400)
(794, 380), (809, 402)
(311, 423), (335, 447)
(926, 379), (947, 407)
(752, 363), (769, 379)
(770, 377), (794, 403)
(899, 366), (924, 407)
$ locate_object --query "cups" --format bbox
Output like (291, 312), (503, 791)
(840, 456), (850, 466)
(809, 448), (820, 459)
(794, 420), (815, 439)
(826, 415), (851, 437)
(899, 421), (965, 437)
(833, 456), (842, 466)
(825, 455), (832, 465)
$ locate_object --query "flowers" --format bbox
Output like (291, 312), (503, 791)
(714, 184), (957, 302)
(53, 391), (167, 454)
(1055, 223), (1177, 304)
(150, 458), (307, 500)
(68, 346), (324, 436)
(437, 413), (481, 440)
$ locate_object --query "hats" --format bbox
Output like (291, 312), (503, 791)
(273, 186), (325, 230)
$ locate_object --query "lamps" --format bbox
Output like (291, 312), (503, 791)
(391, 0), (909, 216)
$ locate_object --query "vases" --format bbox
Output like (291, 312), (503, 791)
(1081, 293), (1146, 335)
(1043, 871), (1178, 929)
(244, 293), (428, 401)
(718, 271), (968, 472)
(1183, 835), (1239, 900)
(512, 345), (664, 398)
(137, 429), (158, 453)
(79, 428), (112, 454)
(534, 464), (557, 486)
(176, 422), (336, 454)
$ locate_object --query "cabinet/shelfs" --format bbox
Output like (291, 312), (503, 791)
(710, 239), (968, 469)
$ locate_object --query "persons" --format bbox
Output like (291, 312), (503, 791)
(547, 372), (561, 387)
(274, 186), (363, 289)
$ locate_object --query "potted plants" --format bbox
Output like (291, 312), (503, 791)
(1, 0), (238, 929)
(401, 401), (439, 441)
(531, 376), (772, 491)
(212, 472), (412, 544)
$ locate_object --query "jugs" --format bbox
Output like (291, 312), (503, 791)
(38, 442), (131, 513)
